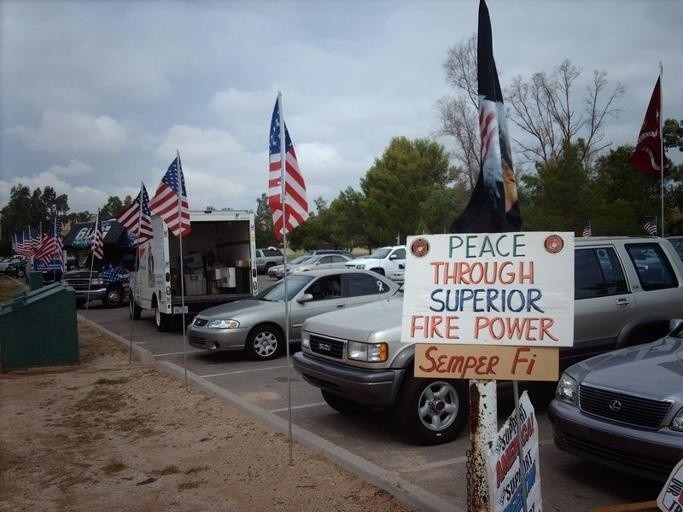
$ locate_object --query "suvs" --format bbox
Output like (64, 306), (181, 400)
(292, 235), (683, 444)
(347, 245), (405, 278)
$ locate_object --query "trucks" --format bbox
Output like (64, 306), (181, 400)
(129, 209), (258, 329)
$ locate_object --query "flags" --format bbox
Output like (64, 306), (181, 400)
(267, 95), (310, 243)
(11, 214), (63, 272)
(450, 1), (526, 236)
(581, 220), (593, 238)
(628, 76), (672, 178)
(89, 213), (106, 262)
(149, 159), (190, 240)
(641, 217), (658, 237)
(114, 186), (155, 246)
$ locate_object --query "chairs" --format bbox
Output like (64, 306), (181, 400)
(324, 281), (341, 299)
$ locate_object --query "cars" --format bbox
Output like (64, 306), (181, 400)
(551, 317), (683, 477)
(256, 247), (351, 279)
(1, 252), (131, 309)
(179, 269), (402, 355)
